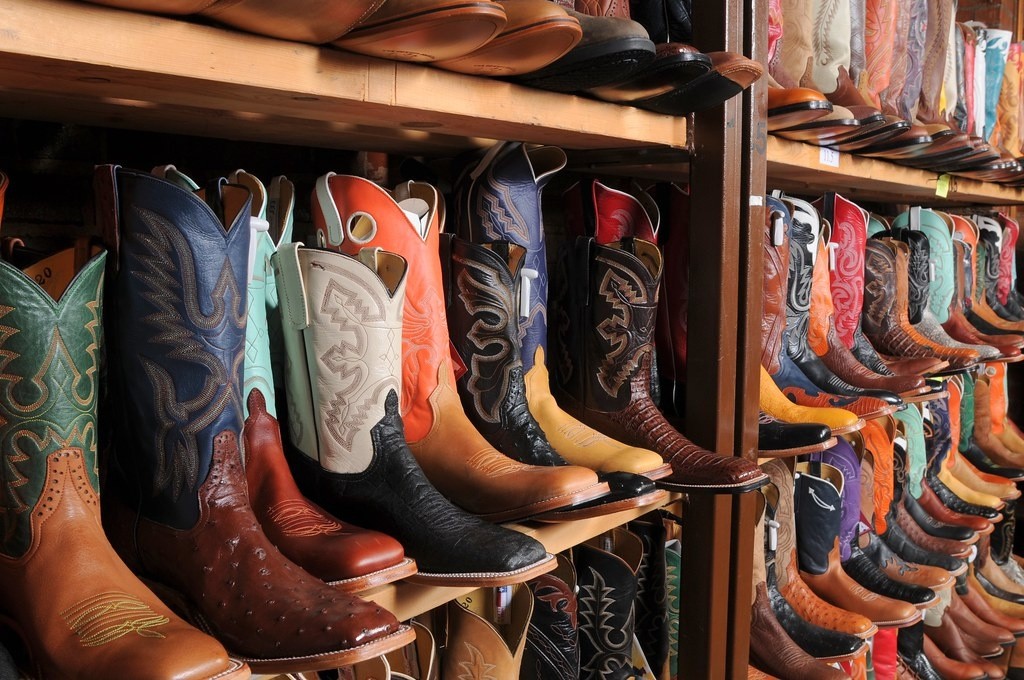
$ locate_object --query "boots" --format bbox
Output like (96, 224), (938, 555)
(748, 1), (1023, 680)
(2, 142), (758, 680)
(96, 0), (764, 125)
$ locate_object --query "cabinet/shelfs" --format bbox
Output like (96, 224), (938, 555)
(1, 0), (1024, 680)
